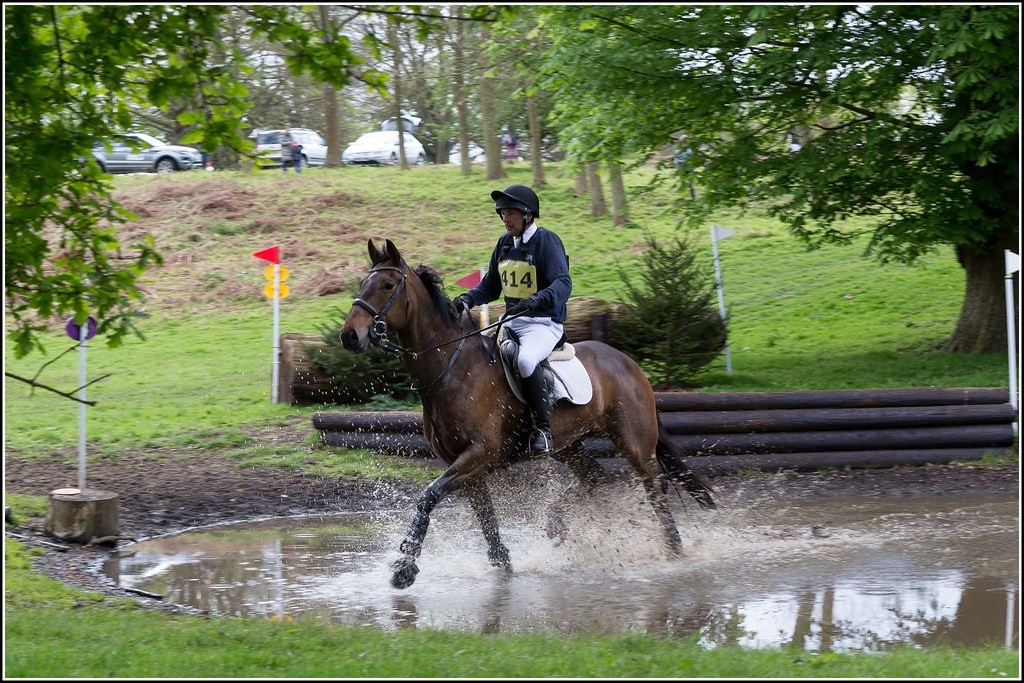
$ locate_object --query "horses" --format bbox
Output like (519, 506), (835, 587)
(340, 237), (719, 589)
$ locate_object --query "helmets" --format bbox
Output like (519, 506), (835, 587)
(491, 193), (539, 212)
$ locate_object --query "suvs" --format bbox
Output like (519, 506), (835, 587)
(257, 126), (330, 167)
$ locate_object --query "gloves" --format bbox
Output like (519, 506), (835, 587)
(453, 293), (475, 313)
(519, 294), (540, 315)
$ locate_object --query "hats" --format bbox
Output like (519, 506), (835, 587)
(491, 184), (539, 218)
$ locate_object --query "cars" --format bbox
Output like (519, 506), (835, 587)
(89, 131), (202, 173)
(342, 130), (427, 167)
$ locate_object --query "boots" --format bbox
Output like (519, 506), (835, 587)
(521, 363), (554, 451)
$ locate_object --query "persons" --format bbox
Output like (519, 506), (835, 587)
(279, 126), (300, 172)
(452, 185), (572, 448)
